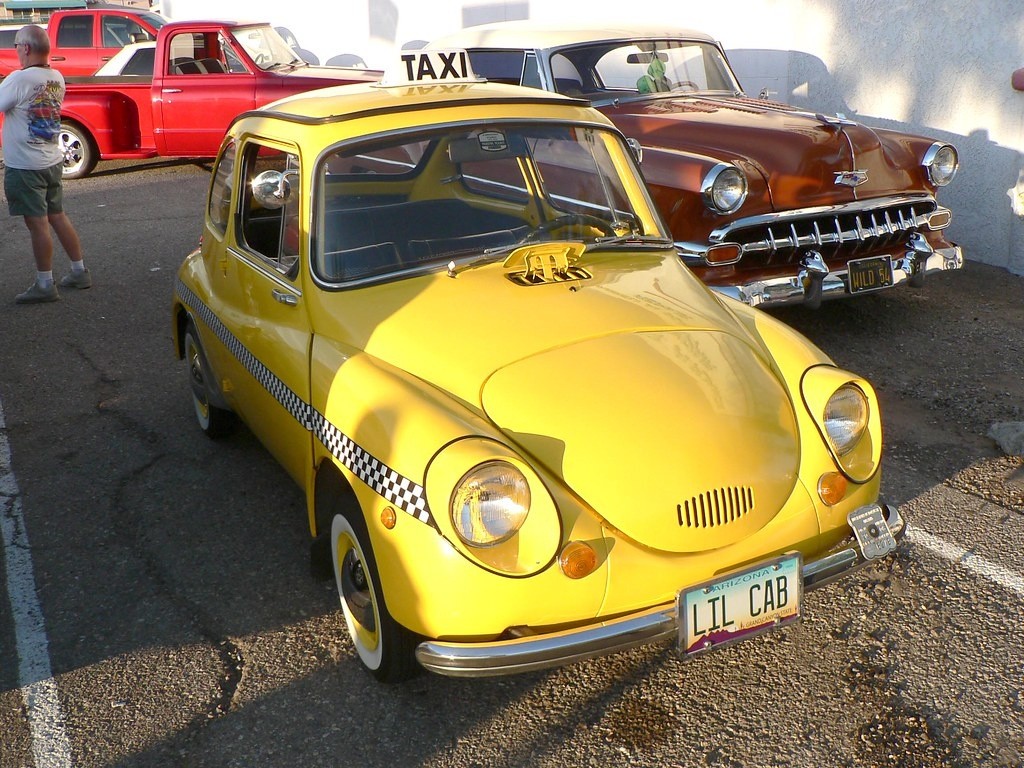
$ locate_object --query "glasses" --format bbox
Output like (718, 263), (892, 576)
(15, 42), (22, 48)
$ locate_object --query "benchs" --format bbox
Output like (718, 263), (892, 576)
(174, 57), (228, 75)
(215, 198), (485, 267)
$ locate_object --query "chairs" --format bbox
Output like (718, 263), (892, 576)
(410, 224), (539, 262)
(555, 78), (586, 95)
(323, 241), (405, 279)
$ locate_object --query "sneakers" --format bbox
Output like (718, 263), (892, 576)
(15, 282), (60, 304)
(58, 268), (93, 289)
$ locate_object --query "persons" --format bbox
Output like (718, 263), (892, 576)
(0, 25), (92, 304)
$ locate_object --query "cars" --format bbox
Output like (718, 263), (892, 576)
(292, 20), (966, 307)
(91, 43), (250, 75)
(171, 74), (909, 685)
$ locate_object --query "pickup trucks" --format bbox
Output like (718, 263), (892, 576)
(0, 10), (169, 75)
(1, 21), (385, 178)
